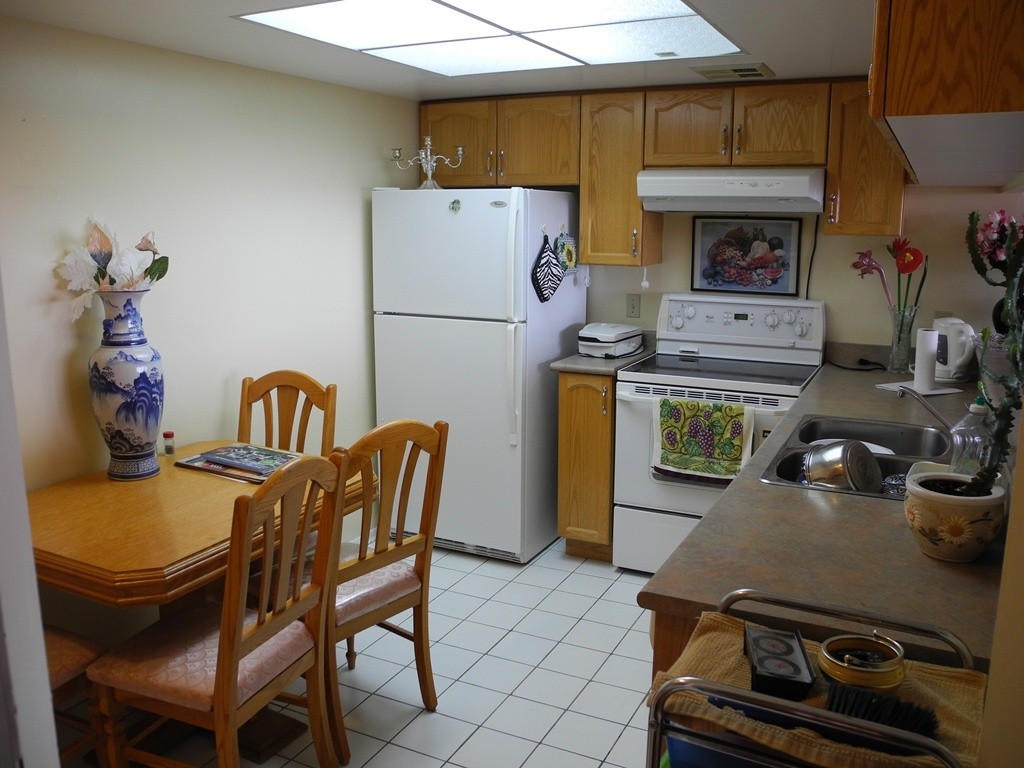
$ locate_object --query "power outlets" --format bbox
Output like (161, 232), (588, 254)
(624, 293), (642, 319)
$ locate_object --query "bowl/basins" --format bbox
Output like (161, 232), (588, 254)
(904, 471), (1006, 563)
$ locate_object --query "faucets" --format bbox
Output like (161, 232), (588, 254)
(896, 384), (952, 429)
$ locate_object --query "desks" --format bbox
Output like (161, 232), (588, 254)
(27, 438), (380, 768)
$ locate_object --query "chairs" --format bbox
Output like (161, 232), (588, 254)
(236, 368), (341, 456)
(323, 416), (451, 765)
(83, 446), (341, 768)
(39, 618), (107, 768)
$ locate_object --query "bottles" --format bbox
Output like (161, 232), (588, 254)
(162, 431), (176, 457)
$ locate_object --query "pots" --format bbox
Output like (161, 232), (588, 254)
(578, 321), (644, 361)
(800, 439), (883, 494)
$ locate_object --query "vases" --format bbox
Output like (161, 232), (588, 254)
(85, 286), (168, 485)
(971, 331), (1020, 410)
(901, 469), (1008, 567)
(884, 304), (919, 375)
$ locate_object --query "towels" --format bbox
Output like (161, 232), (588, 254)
(650, 396), (756, 481)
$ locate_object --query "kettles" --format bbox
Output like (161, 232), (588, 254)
(932, 316), (976, 378)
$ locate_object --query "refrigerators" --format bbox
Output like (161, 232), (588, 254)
(369, 184), (589, 562)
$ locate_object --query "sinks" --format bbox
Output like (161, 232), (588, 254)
(786, 412), (952, 464)
(757, 444), (944, 502)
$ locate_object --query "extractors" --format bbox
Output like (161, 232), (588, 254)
(634, 167), (826, 216)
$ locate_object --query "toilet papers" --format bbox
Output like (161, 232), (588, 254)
(914, 328), (939, 390)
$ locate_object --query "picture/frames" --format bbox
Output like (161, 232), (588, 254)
(687, 213), (805, 301)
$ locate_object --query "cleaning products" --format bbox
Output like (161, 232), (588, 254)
(949, 394), (993, 473)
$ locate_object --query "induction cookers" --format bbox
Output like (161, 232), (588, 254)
(618, 289), (828, 398)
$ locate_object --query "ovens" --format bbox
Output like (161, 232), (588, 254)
(610, 383), (799, 576)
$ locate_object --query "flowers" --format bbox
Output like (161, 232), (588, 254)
(54, 212), (171, 329)
(846, 236), (930, 346)
(955, 207), (1024, 495)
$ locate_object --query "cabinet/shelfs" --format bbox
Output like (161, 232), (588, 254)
(578, 91), (664, 270)
(533, 372), (615, 567)
(413, 95), (581, 189)
(821, 80), (907, 240)
(643, 81), (833, 169)
(866, 0), (1024, 189)
